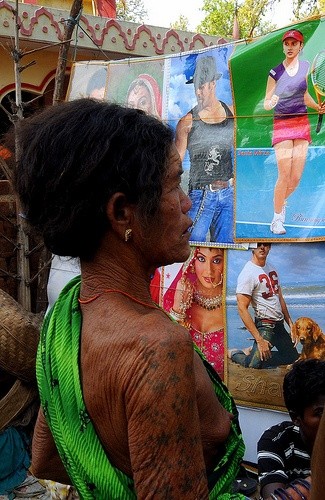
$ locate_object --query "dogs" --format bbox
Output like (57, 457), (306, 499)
(286, 317), (325, 371)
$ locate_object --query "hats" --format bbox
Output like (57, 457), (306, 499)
(282, 30), (303, 42)
(185, 57), (222, 85)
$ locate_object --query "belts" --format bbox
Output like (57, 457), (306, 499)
(197, 181), (228, 192)
(261, 318), (284, 324)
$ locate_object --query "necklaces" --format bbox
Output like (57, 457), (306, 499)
(77, 289), (165, 317)
(192, 276), (223, 311)
(284, 57), (298, 74)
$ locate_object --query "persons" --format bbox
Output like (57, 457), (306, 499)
(171, 56), (237, 243)
(161, 245), (226, 384)
(86, 69), (108, 102)
(125, 73), (166, 123)
(263, 28), (325, 235)
(13, 98), (256, 500)
(309, 409), (325, 500)
(226, 241), (302, 370)
(255, 356), (325, 500)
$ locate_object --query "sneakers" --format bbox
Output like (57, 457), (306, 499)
(282, 205), (285, 222)
(270, 220), (286, 234)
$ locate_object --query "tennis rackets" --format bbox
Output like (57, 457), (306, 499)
(311, 50), (325, 133)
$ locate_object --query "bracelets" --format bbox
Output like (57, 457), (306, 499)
(270, 478), (313, 500)
(268, 100), (277, 108)
(168, 277), (194, 323)
